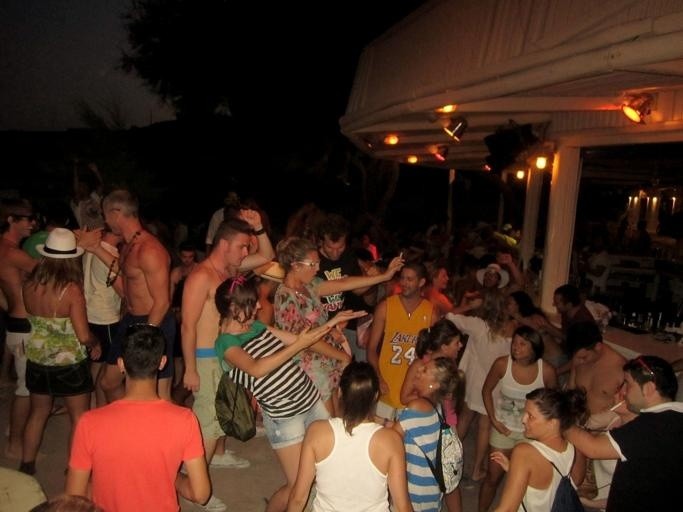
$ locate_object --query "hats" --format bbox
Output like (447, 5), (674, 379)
(474, 263), (510, 288)
(35, 227), (85, 258)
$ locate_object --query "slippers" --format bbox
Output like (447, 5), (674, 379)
(51, 401), (66, 415)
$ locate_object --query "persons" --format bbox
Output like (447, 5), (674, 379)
(0, 186), (683, 512)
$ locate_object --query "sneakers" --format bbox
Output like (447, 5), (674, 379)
(208, 448), (250, 470)
(201, 494), (227, 511)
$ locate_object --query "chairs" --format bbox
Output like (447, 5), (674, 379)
(587, 264), (613, 296)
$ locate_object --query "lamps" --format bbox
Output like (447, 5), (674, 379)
(437, 146), (450, 162)
(482, 122), (550, 181)
(444, 115), (470, 142)
(621, 94), (659, 128)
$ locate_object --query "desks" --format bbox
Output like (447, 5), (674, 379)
(608, 252), (656, 271)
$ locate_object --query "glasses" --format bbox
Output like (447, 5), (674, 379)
(296, 260), (318, 267)
(14, 213), (37, 222)
(635, 353), (657, 383)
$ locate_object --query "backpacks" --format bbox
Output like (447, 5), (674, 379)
(411, 396), (465, 495)
(522, 445), (585, 512)
(214, 371), (259, 442)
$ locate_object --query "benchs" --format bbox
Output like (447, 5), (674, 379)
(614, 265), (661, 304)
(538, 311), (682, 375)
